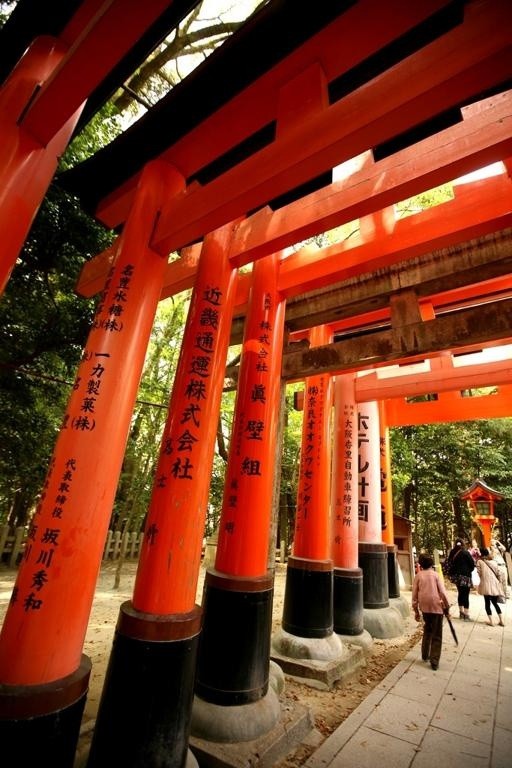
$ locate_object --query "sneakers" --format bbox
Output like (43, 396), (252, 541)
(464, 613), (473, 622)
(459, 611), (465, 618)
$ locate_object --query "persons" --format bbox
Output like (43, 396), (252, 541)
(411, 536), (512, 670)
